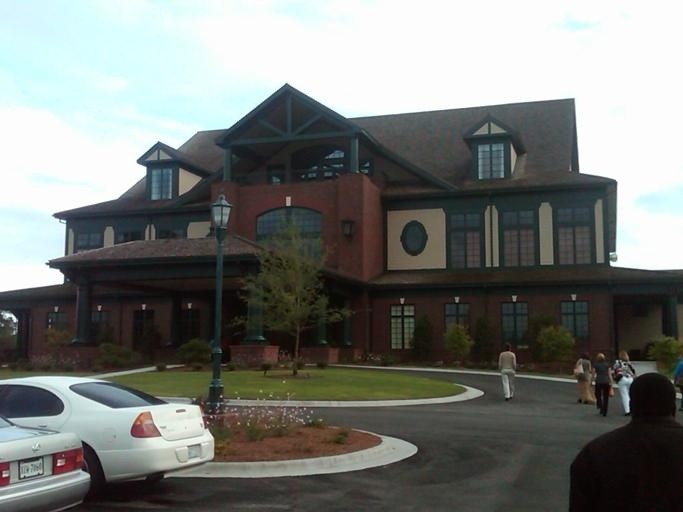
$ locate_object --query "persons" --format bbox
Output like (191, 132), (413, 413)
(672, 358), (682, 410)
(612, 350), (635, 413)
(497, 342), (517, 399)
(574, 352), (593, 406)
(589, 353), (611, 413)
(564, 373), (683, 510)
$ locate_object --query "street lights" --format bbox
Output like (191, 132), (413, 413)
(202, 194), (235, 432)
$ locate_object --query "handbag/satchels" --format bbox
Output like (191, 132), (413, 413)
(615, 367), (623, 384)
(573, 364), (585, 377)
(610, 386), (614, 396)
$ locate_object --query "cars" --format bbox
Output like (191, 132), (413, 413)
(0, 412), (91, 512)
(0, 375), (215, 503)
